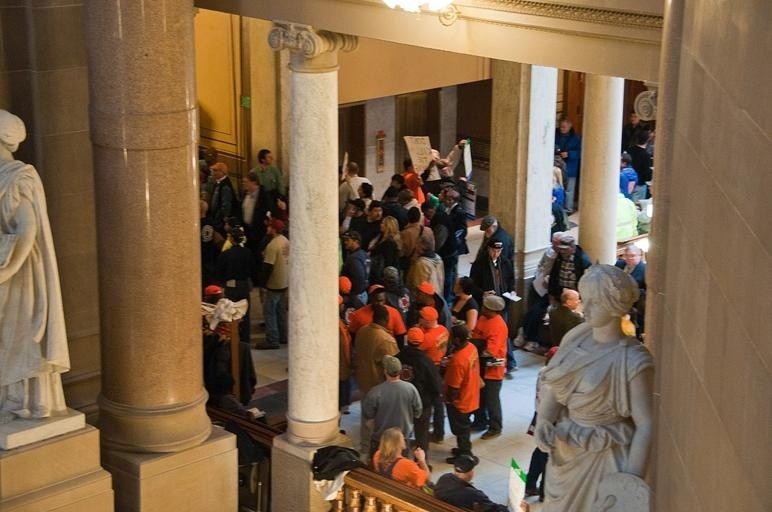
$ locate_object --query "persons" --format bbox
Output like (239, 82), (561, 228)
(617, 187), (639, 247)
(362, 354), (423, 463)
(431, 139), (470, 181)
(513, 231), (568, 351)
(427, 180), (450, 213)
(0, 107), (72, 425)
(404, 235), (446, 295)
(551, 167), (568, 210)
(249, 150), (285, 197)
(549, 236), (592, 298)
(480, 215), (513, 259)
(432, 453), (510, 512)
(340, 230), (371, 303)
(450, 277), (480, 332)
(554, 118), (581, 212)
(349, 306), (400, 404)
(469, 240), (518, 372)
(437, 190), (466, 233)
(619, 153), (639, 198)
(548, 289), (586, 346)
(254, 219), (290, 352)
(441, 327), (482, 456)
(397, 328), (444, 459)
(473, 296), (509, 441)
(524, 344), (558, 504)
(406, 282), (449, 330)
(340, 161), (375, 209)
(420, 199), (457, 279)
(348, 182), (383, 210)
(372, 427), (431, 489)
(341, 198), (367, 235)
(382, 173), (407, 199)
(623, 113), (646, 146)
(631, 131), (653, 198)
(348, 284), (406, 348)
(529, 260), (656, 510)
(206, 162), (240, 230)
(396, 192), (424, 226)
(372, 215), (401, 268)
(401, 157), (435, 206)
(237, 173), (275, 235)
(220, 228), (252, 313)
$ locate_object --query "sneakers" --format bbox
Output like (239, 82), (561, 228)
(513, 334), (526, 347)
(525, 340), (538, 351)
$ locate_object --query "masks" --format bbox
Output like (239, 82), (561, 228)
(340, 230), (361, 241)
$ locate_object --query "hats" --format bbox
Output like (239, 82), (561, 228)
(436, 158), (453, 167)
(383, 354), (403, 377)
(487, 238), (503, 249)
(339, 276), (352, 294)
(479, 215), (498, 231)
(347, 199), (366, 210)
(369, 284), (383, 293)
(456, 453), (479, 472)
(416, 281), (436, 295)
(384, 266), (399, 283)
(483, 295), (505, 312)
(420, 306), (439, 321)
(210, 162), (228, 176)
(265, 218), (285, 232)
(406, 327), (425, 344)
(223, 216), (241, 228)
(556, 234), (575, 249)
